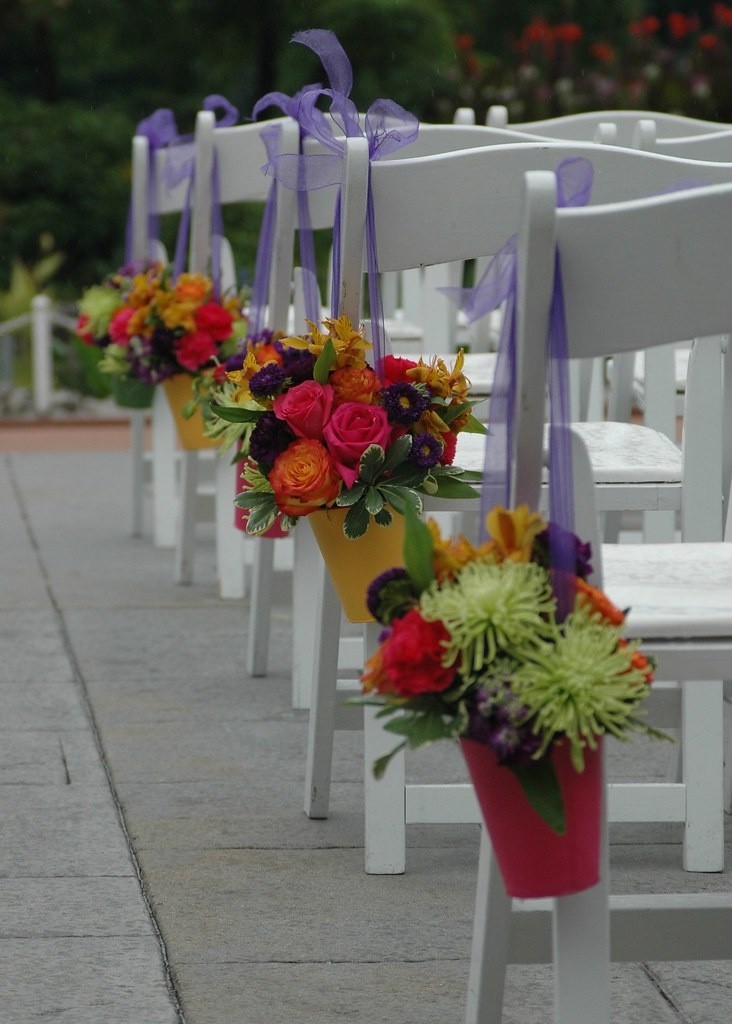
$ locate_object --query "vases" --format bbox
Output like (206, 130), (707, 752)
(457, 727), (606, 898)
(163, 370), (227, 452)
(305, 502), (411, 625)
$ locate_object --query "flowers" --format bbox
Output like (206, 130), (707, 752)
(356, 501), (678, 841)
(65, 254), (493, 541)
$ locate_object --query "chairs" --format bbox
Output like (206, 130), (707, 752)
(132, 107), (731, 1024)
